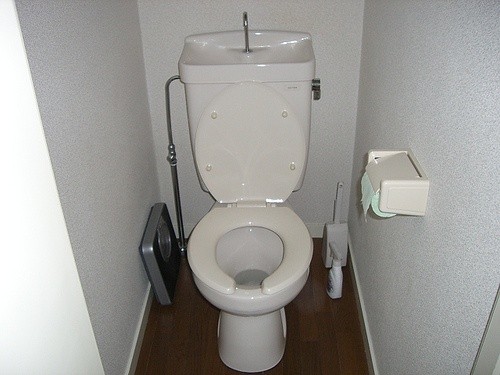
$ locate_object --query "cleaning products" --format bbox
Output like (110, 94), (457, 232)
(324, 240), (346, 300)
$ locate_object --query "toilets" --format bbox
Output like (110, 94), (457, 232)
(176, 28), (324, 374)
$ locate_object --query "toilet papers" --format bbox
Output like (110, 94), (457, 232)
(358, 171), (401, 220)
